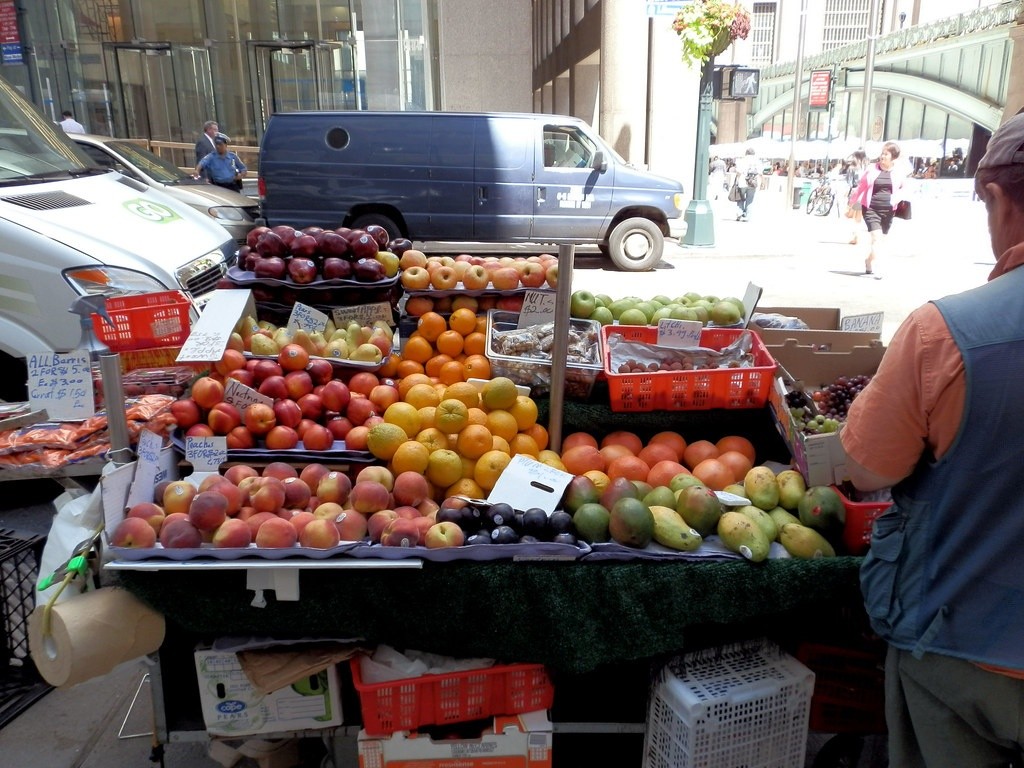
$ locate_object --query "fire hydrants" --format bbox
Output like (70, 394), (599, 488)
(793, 186), (804, 208)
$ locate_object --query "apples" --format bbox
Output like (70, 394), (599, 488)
(573, 290), (747, 327)
(235, 225), (558, 316)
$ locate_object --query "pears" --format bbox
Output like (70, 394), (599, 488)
(225, 315), (394, 364)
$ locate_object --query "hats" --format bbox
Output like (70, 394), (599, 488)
(213, 132), (231, 145)
(973, 110), (1024, 202)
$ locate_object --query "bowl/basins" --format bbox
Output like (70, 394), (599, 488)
(485, 307), (605, 400)
(123, 366), (196, 398)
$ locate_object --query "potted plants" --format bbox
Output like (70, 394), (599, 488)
(672, 0), (751, 69)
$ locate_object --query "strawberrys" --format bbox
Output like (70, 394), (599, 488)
(94, 366), (195, 408)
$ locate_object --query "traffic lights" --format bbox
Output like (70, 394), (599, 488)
(729, 68), (760, 98)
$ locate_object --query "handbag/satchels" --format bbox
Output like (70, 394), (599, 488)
(893, 200), (912, 220)
(728, 174), (742, 202)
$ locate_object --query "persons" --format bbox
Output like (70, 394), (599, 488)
(839, 106), (1023, 767)
(60, 111), (85, 133)
(736, 148), (759, 220)
(844, 142), (906, 279)
(774, 162), (824, 177)
(917, 157), (958, 178)
(709, 156), (726, 201)
(195, 120), (219, 183)
(192, 132), (247, 193)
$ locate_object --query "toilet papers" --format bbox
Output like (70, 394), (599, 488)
(29, 583), (166, 687)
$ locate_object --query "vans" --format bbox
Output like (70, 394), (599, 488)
(259, 111), (685, 272)
(0, 66), (260, 402)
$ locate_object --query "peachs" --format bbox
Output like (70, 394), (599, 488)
(171, 344), (401, 453)
(110, 462), (463, 548)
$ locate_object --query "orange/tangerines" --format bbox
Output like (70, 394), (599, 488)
(365, 308), (568, 505)
(560, 427), (756, 492)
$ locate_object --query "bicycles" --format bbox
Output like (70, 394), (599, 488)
(807, 177), (835, 216)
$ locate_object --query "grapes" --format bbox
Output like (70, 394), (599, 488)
(785, 372), (876, 438)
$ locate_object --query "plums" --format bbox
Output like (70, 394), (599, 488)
(434, 502), (580, 550)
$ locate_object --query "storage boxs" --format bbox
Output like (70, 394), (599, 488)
(483, 305), (893, 550)
(643, 640), (816, 768)
(349, 645), (553, 735)
(357, 710), (552, 768)
(194, 640), (342, 736)
(790, 641), (885, 734)
(89, 290), (190, 353)
(0, 499), (63, 726)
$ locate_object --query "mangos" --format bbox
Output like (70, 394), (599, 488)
(562, 465), (846, 561)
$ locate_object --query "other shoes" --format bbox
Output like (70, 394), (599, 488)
(736, 212), (748, 223)
(873, 269), (882, 279)
(864, 258), (873, 274)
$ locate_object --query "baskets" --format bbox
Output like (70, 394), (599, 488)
(601, 324), (779, 414)
(642, 635), (816, 768)
(830, 484), (895, 556)
(89, 289), (192, 353)
(351, 655), (557, 737)
